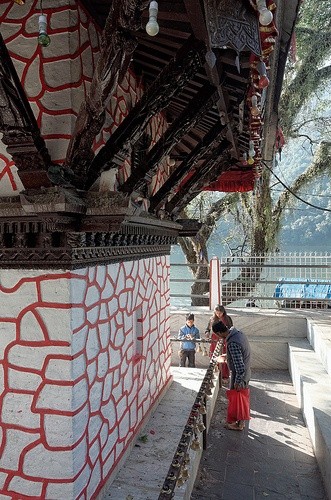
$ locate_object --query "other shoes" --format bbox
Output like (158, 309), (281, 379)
(226, 422), (245, 430)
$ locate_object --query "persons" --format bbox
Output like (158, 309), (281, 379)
(204, 304), (233, 381)
(178, 313), (200, 368)
(212, 321), (251, 431)
